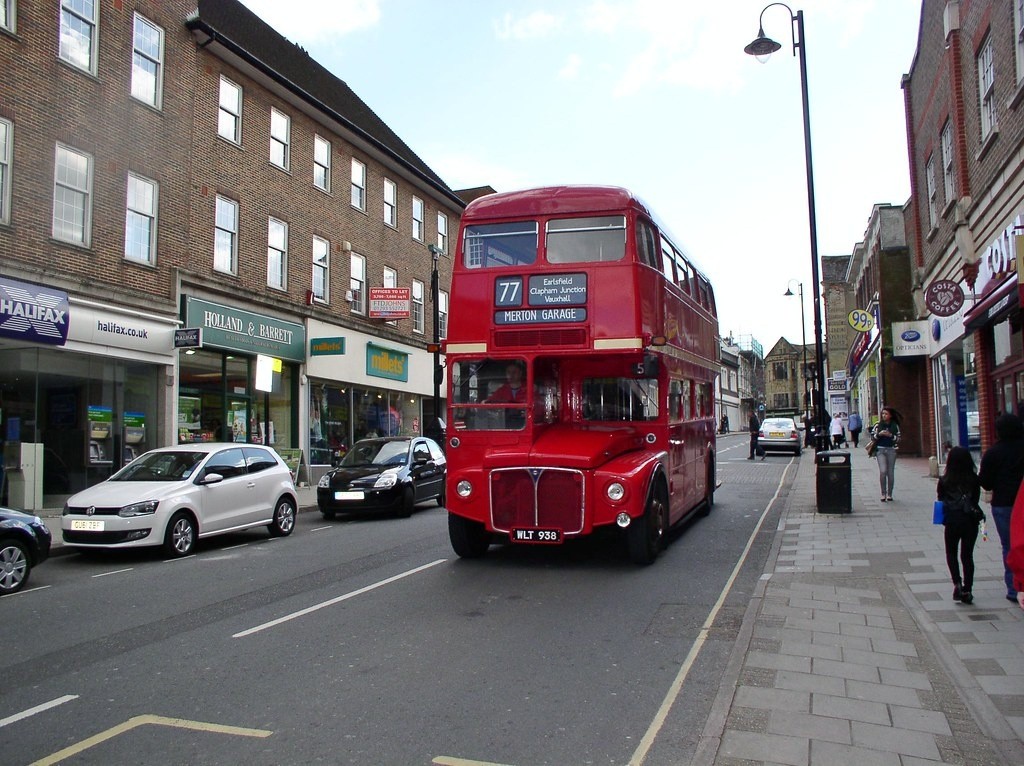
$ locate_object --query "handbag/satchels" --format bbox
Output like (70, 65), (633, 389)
(856, 427), (862, 433)
(865, 438), (877, 457)
(933, 501), (944, 524)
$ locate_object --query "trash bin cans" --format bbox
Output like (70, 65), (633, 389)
(817, 450), (851, 514)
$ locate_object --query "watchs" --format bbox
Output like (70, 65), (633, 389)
(889, 433), (892, 437)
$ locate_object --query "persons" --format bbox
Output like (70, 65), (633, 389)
(481, 364), (541, 424)
(801, 410), (862, 450)
(334, 427), (378, 464)
(308, 398), (324, 455)
(870, 407), (904, 501)
(747, 410), (767, 460)
(937, 412), (1024, 608)
(191, 400), (199, 423)
(719, 414), (729, 434)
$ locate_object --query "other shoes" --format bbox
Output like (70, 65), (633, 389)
(881, 496), (886, 501)
(747, 456), (754, 460)
(762, 453), (767, 460)
(887, 496), (892, 501)
(953, 582), (973, 604)
(1006, 594), (1018, 602)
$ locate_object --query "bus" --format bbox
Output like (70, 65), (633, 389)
(426, 185), (720, 566)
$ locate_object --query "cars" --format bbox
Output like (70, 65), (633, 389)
(316, 436), (447, 517)
(0, 507), (52, 595)
(61, 442), (299, 559)
(755, 418), (801, 456)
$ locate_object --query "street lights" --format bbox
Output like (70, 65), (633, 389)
(784, 279), (809, 419)
(744, 2), (828, 462)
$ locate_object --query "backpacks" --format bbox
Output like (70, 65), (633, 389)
(939, 476), (986, 524)
